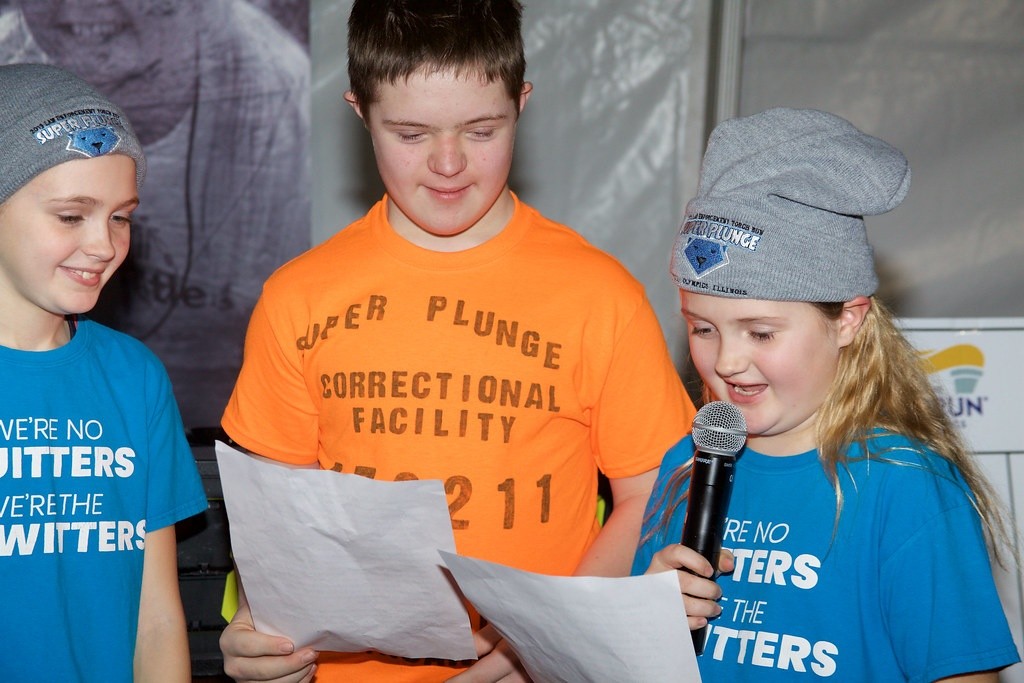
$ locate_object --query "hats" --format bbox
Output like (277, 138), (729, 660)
(0, 62), (148, 204)
(669, 107), (914, 300)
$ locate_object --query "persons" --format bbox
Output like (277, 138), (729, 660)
(1, 0), (317, 607)
(622, 107), (1023, 683)
(216, 4), (700, 682)
(0, 63), (210, 683)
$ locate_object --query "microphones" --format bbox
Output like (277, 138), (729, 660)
(675, 401), (748, 656)
